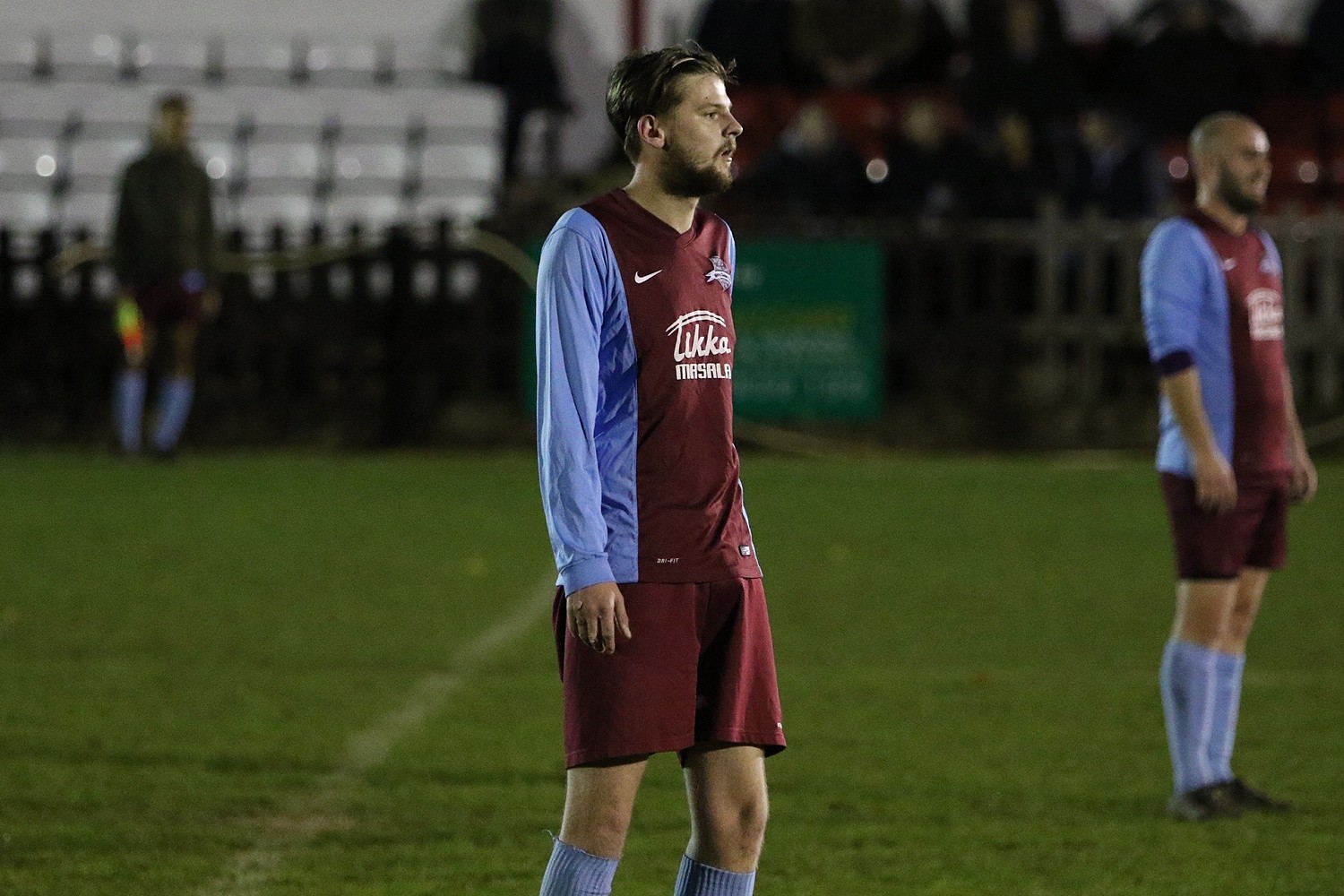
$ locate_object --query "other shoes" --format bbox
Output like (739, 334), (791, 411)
(1220, 777), (1293, 816)
(1169, 785), (1247, 826)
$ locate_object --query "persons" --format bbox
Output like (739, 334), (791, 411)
(535, 45), (789, 896)
(110, 95), (223, 458)
(1138, 116), (1317, 822)
(765, 95), (1173, 392)
